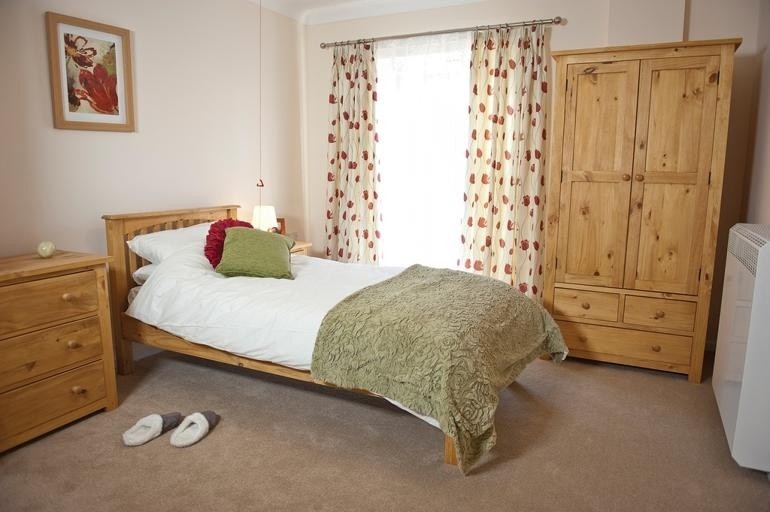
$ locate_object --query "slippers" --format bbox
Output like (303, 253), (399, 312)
(122, 411), (181, 446)
(170, 410), (217, 448)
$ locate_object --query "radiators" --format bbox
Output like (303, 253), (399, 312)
(712, 222), (770, 473)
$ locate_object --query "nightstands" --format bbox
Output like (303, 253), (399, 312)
(291, 241), (312, 256)
(0, 251), (115, 454)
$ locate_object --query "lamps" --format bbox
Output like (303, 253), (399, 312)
(252, 205), (278, 233)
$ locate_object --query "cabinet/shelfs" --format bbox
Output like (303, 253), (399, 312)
(539, 38), (742, 384)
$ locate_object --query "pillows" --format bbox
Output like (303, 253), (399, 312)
(126, 220), (296, 285)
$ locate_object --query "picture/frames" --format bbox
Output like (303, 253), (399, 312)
(46, 12), (135, 132)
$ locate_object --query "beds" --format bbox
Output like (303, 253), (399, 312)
(102, 205), (570, 475)
